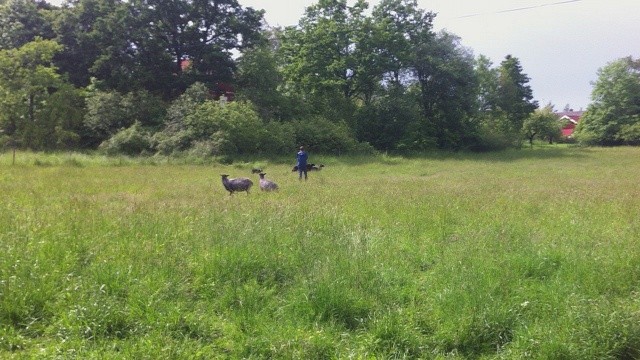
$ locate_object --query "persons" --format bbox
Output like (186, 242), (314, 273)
(296, 146), (308, 181)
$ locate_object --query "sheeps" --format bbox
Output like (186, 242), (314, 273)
(221, 174), (253, 195)
(252, 168), (263, 174)
(258, 173), (278, 191)
(292, 163), (324, 172)
